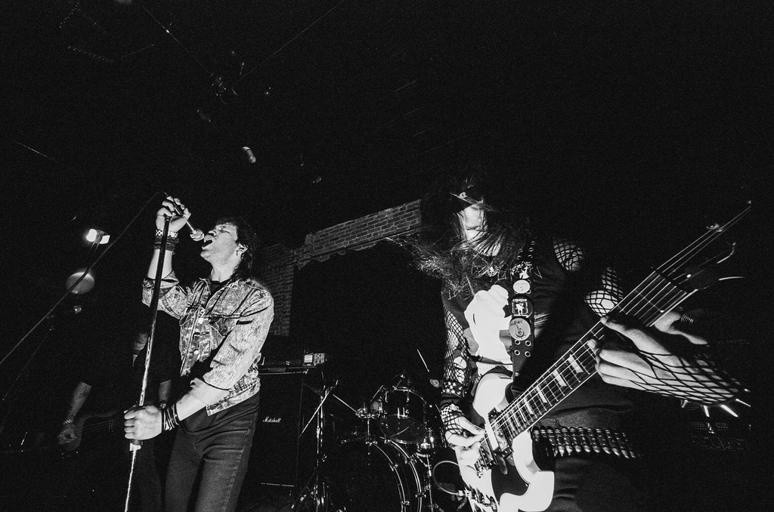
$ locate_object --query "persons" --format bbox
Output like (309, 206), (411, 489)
(59, 326), (170, 511)
(418, 142), (773, 511)
(124, 192), (274, 512)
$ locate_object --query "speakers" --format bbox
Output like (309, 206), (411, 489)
(247, 366), (320, 499)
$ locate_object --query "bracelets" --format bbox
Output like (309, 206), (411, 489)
(153, 228), (178, 253)
(159, 402), (182, 432)
(439, 401), (463, 422)
(61, 416), (77, 425)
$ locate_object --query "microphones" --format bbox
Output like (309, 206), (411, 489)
(164, 192), (205, 243)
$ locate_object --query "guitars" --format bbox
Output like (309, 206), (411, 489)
(55, 407), (130, 466)
(454, 198), (753, 512)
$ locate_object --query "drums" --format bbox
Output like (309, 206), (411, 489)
(312, 430), (423, 512)
(369, 384), (428, 445)
(405, 401), (447, 454)
